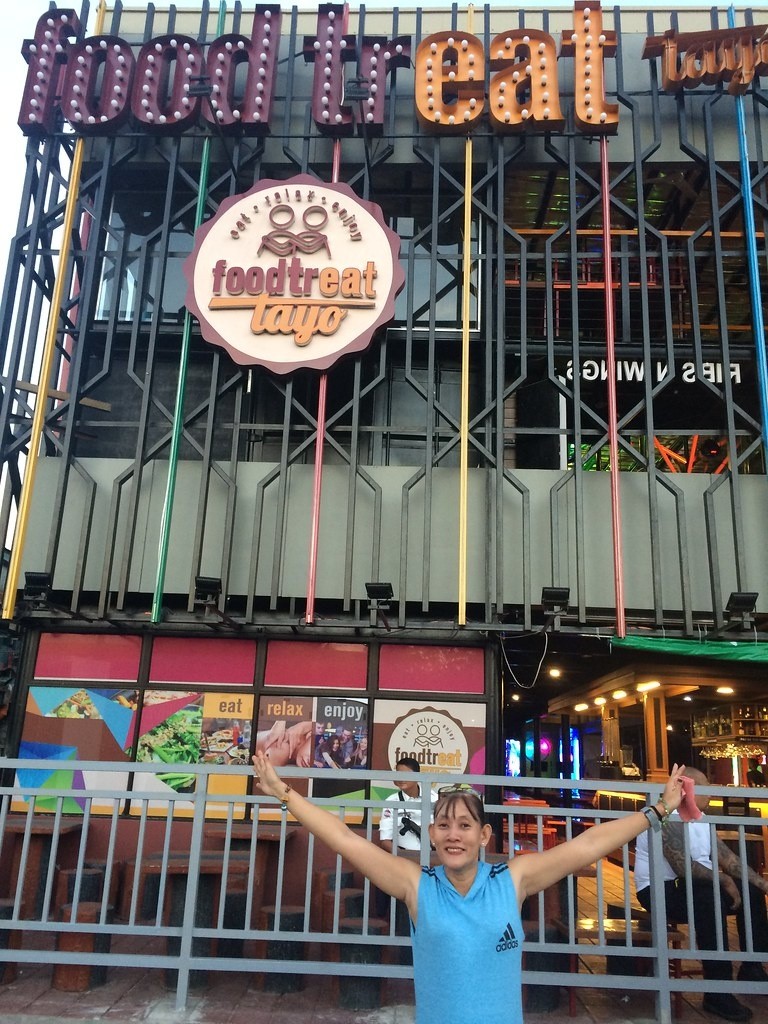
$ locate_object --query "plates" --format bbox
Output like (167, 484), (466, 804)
(228, 745), (250, 758)
(200, 755), (225, 764)
(212, 730), (233, 742)
(199, 742), (233, 752)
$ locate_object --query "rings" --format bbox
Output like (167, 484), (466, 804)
(257, 776), (260, 780)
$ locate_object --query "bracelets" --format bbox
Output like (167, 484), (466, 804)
(641, 797), (670, 830)
(281, 785), (293, 812)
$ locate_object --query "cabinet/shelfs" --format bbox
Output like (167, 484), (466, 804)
(688, 701), (768, 747)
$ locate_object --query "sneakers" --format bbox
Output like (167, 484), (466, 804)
(703, 992), (753, 1020)
(737, 962), (768, 982)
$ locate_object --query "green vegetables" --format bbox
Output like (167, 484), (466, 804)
(131, 710), (203, 790)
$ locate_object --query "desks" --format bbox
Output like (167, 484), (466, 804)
(393, 845), (598, 924)
(204, 822), (298, 943)
(144, 849), (255, 997)
(2, 816), (86, 935)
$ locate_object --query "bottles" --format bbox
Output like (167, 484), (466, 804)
(763, 705), (768, 720)
(748, 722), (755, 735)
(738, 704), (743, 719)
(739, 722), (745, 735)
(744, 705), (752, 719)
(244, 720), (252, 749)
(233, 725), (240, 746)
(762, 726), (766, 736)
(693, 715), (732, 737)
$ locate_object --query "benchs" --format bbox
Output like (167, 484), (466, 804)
(551, 901), (707, 1019)
(502, 791), (603, 855)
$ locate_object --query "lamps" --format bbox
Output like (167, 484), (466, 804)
(725, 592), (759, 621)
(194, 576), (222, 605)
(716, 686), (733, 694)
(24, 571), (53, 601)
(363, 582), (394, 609)
(541, 587), (570, 616)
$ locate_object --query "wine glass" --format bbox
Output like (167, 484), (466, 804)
(699, 745), (764, 761)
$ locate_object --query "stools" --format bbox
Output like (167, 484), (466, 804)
(519, 921), (561, 1014)
(0, 855), (389, 1011)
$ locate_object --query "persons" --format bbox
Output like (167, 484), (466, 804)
(313, 718), (367, 769)
(251, 749), (686, 1024)
(634, 765), (768, 1020)
(257, 720), (312, 767)
(379, 757), (440, 850)
(746, 758), (766, 787)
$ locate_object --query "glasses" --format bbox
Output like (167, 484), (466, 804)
(438, 786), (484, 811)
